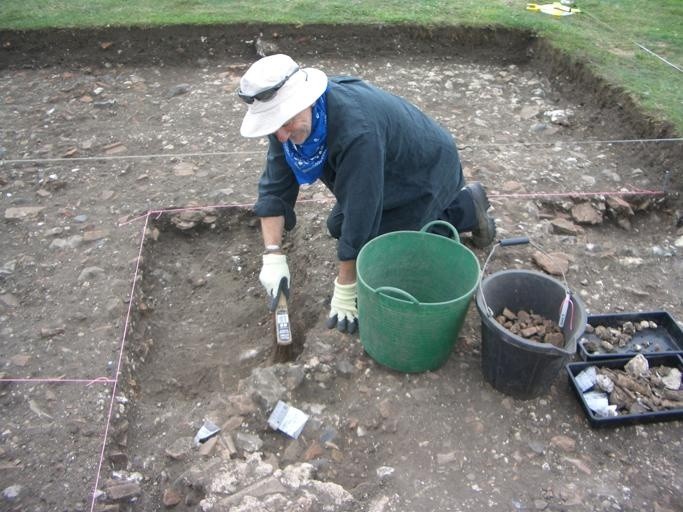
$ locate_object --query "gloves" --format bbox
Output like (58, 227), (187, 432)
(327, 274), (360, 333)
(257, 245), (293, 313)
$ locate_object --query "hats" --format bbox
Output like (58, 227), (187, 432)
(236, 53), (329, 139)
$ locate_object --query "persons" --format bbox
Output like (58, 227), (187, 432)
(238, 52), (496, 335)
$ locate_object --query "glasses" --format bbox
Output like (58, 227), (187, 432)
(238, 68), (301, 106)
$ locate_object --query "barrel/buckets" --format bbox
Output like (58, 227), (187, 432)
(355, 219), (482, 370)
(473, 236), (588, 398)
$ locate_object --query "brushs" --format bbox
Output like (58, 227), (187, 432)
(272, 290), (293, 362)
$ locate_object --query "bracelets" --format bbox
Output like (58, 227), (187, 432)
(262, 246), (284, 255)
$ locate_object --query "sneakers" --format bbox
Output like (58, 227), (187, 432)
(461, 181), (498, 250)
